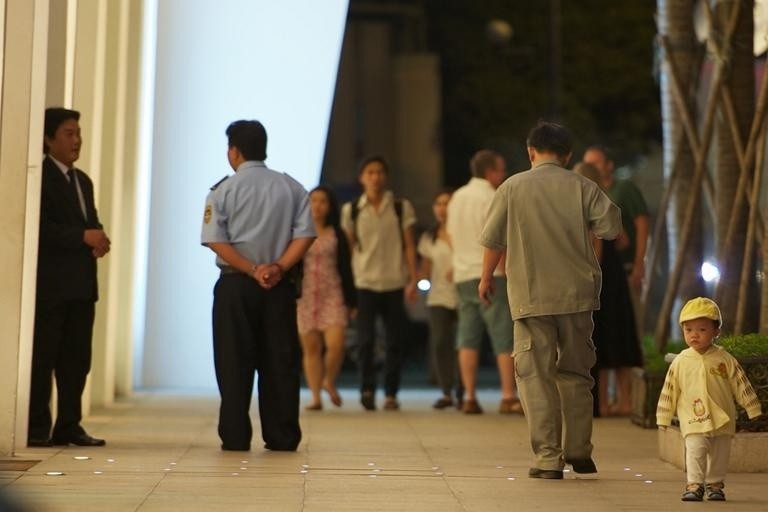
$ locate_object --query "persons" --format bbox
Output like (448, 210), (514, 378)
(417, 190), (465, 410)
(19, 107), (107, 448)
(476, 120), (625, 480)
(198, 118), (317, 453)
(340, 153), (419, 408)
(570, 145), (648, 416)
(656, 297), (764, 502)
(446, 149), (525, 418)
(293, 184), (358, 407)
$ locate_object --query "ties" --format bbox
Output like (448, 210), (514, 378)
(67, 170), (84, 221)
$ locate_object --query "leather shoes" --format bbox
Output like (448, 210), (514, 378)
(53, 426), (105, 447)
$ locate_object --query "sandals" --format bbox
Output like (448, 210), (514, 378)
(681, 484), (704, 501)
(705, 482), (726, 501)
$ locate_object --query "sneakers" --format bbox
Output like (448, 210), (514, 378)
(566, 458), (596, 473)
(307, 382), (517, 413)
(529, 469), (563, 479)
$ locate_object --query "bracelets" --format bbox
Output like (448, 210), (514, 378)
(249, 264), (259, 278)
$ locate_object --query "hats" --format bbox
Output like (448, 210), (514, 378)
(679, 297), (723, 328)
